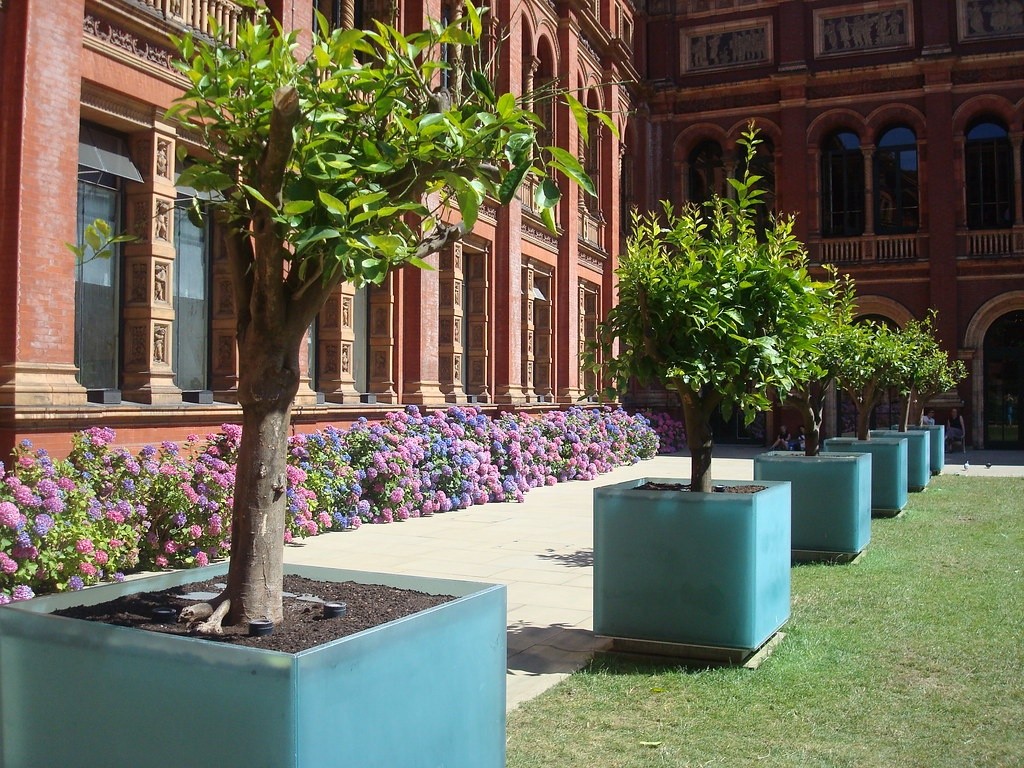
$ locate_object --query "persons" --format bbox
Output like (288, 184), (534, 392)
(342, 346), (348, 372)
(154, 265), (167, 300)
(155, 205), (168, 241)
(824, 11), (902, 50)
(343, 300), (349, 326)
(1004, 393), (1013, 428)
(944, 408), (965, 454)
(154, 328), (167, 363)
(158, 141), (168, 177)
(768, 426), (806, 451)
(694, 29), (766, 69)
(968, 0), (1024, 33)
(923, 407), (936, 426)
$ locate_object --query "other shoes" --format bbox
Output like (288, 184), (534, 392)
(949, 450), (952, 454)
(769, 447), (773, 451)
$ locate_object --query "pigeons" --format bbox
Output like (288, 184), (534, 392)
(985, 461), (992, 469)
(964, 461), (970, 470)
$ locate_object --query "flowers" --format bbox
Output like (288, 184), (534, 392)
(0, 403), (687, 606)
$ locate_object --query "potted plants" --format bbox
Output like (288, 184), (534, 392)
(890, 349), (970, 472)
(753, 262), (882, 555)
(869, 308), (950, 487)
(575, 117), (861, 651)
(0, 0), (620, 768)
(823, 319), (909, 508)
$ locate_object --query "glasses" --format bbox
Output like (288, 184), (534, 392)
(930, 412), (934, 413)
